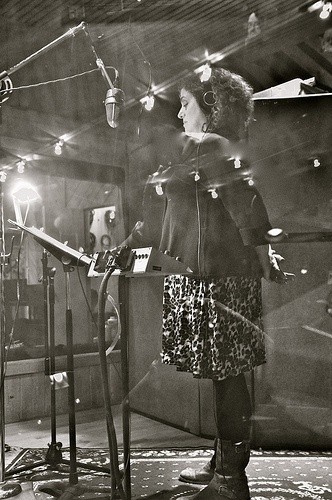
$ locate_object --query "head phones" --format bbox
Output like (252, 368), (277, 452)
(200, 73), (219, 112)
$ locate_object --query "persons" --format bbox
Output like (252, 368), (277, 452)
(160, 67), (296, 500)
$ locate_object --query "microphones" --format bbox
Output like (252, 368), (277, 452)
(105, 88), (125, 129)
(114, 221), (156, 252)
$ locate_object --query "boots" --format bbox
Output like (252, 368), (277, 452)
(179, 438), (218, 484)
(174, 438), (252, 500)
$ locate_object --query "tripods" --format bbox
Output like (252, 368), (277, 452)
(3, 219), (125, 500)
